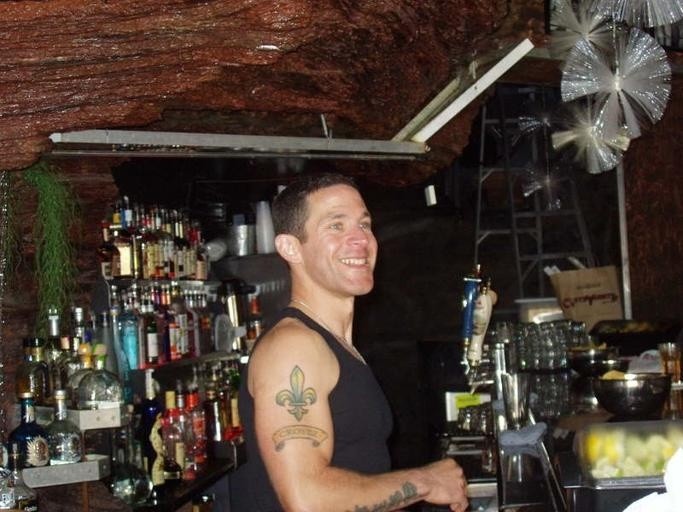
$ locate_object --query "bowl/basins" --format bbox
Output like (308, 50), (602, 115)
(591, 369), (675, 421)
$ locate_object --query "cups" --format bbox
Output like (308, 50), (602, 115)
(500, 372), (531, 432)
(658, 341), (682, 388)
(505, 447), (535, 485)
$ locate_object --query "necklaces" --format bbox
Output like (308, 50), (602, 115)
(290, 297), (364, 362)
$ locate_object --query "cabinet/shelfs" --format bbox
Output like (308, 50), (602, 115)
(91, 260), (234, 512)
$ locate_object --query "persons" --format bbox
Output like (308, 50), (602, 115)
(238, 170), (470, 512)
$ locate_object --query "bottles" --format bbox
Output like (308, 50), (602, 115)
(457, 402), (497, 476)
(0, 191), (267, 512)
(534, 373), (570, 419)
(500, 318), (588, 372)
